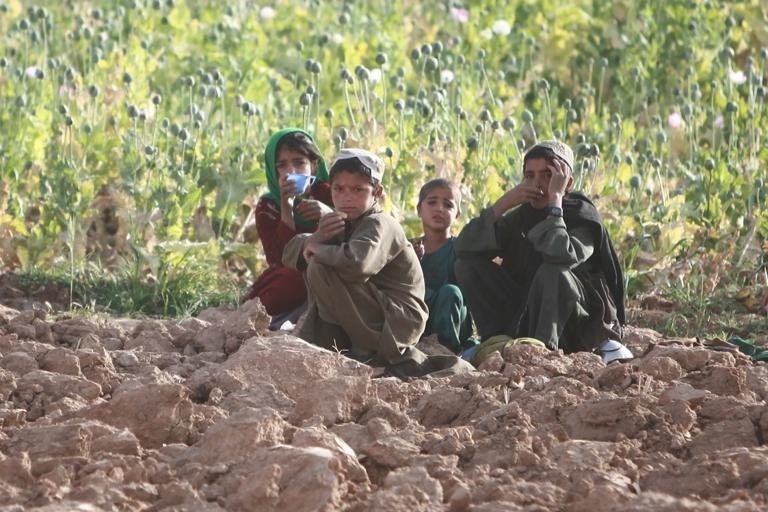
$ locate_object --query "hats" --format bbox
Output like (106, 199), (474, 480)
(330, 148), (385, 183)
(535, 140), (574, 173)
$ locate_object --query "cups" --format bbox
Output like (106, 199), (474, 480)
(285, 173), (316, 194)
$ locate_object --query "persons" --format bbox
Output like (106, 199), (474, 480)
(402, 174), (474, 352)
(453, 135), (626, 360)
(280, 146), (429, 371)
(240, 127), (333, 330)
